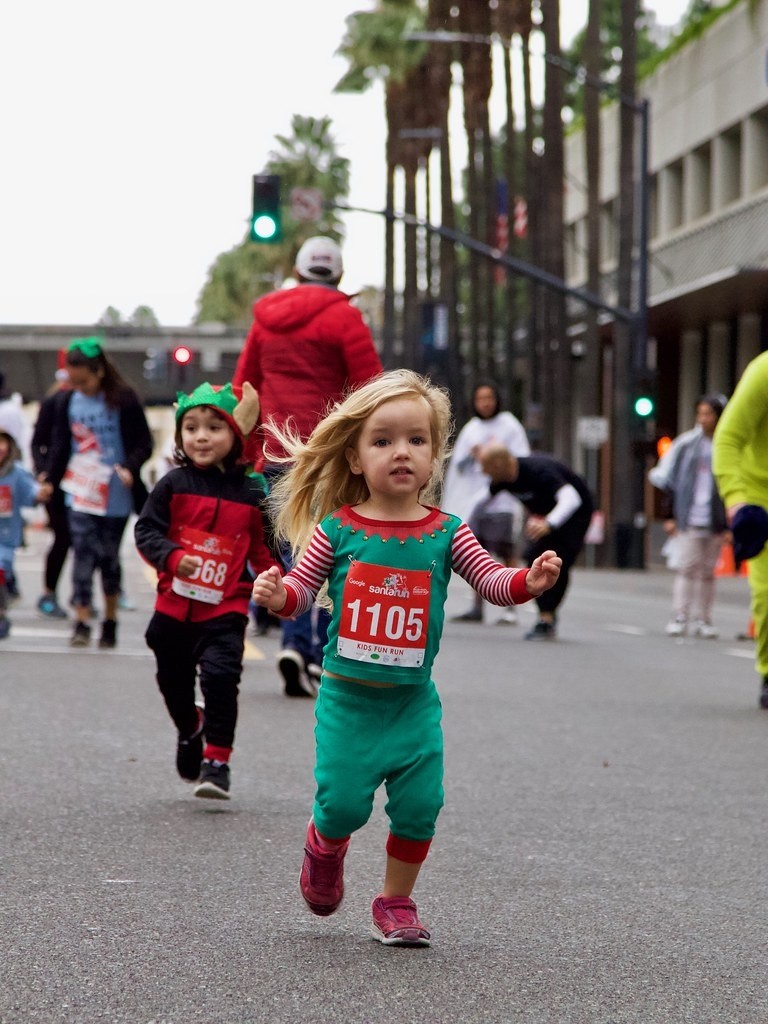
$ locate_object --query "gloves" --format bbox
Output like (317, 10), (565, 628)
(730, 505), (768, 571)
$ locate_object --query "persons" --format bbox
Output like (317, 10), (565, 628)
(32, 337), (154, 645)
(253, 370), (563, 944)
(710, 348), (768, 707)
(479, 443), (595, 642)
(234, 236), (384, 699)
(0, 426), (54, 606)
(441, 380), (531, 626)
(649, 396), (729, 639)
(134, 381), (296, 799)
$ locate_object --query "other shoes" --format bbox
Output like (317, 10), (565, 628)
(308, 664), (322, 682)
(499, 609), (521, 624)
(760, 674), (768, 709)
(452, 604), (483, 622)
(71, 622), (92, 645)
(666, 618), (687, 635)
(695, 620), (719, 637)
(99, 620), (117, 647)
(277, 650), (316, 698)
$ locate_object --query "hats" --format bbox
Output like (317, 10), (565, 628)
(295, 236), (342, 283)
(703, 392), (730, 416)
(0, 391), (45, 460)
(174, 381), (261, 451)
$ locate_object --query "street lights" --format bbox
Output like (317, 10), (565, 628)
(411, 30), (650, 567)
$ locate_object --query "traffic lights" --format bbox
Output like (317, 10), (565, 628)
(251, 175), (281, 242)
(176, 348), (188, 383)
(633, 374), (653, 421)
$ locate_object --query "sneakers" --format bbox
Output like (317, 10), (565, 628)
(299, 813), (351, 917)
(526, 620), (555, 642)
(370, 893), (432, 946)
(194, 760), (231, 799)
(176, 699), (204, 783)
(37, 595), (68, 620)
(71, 593), (98, 618)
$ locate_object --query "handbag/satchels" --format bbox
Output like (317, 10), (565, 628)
(477, 512), (512, 561)
(710, 494), (727, 534)
